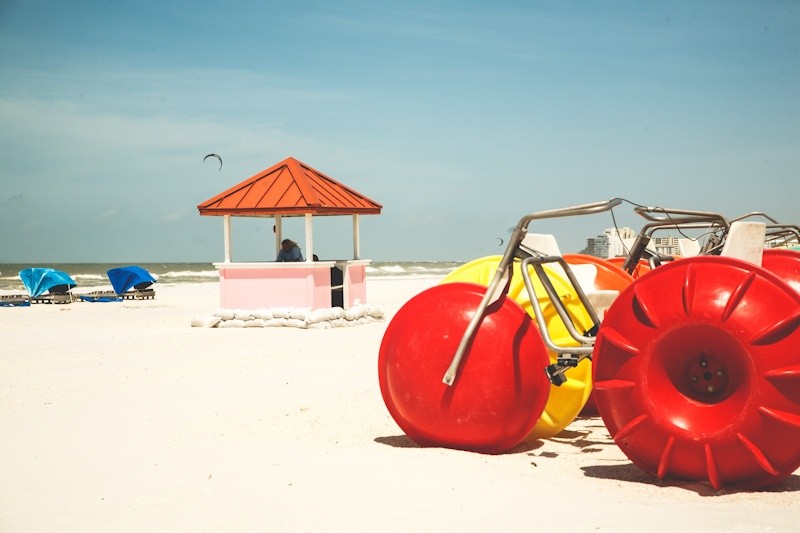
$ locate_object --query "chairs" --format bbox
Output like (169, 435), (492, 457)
(76, 287), (155, 301)
(0, 290), (75, 303)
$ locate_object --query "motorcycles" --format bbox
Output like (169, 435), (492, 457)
(377, 196), (800, 493)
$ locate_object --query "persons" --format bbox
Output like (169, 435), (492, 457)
(277, 239), (304, 262)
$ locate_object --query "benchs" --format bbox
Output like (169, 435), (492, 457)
(719, 221), (768, 270)
(622, 237), (657, 261)
(678, 237), (702, 258)
(517, 230), (622, 327)
